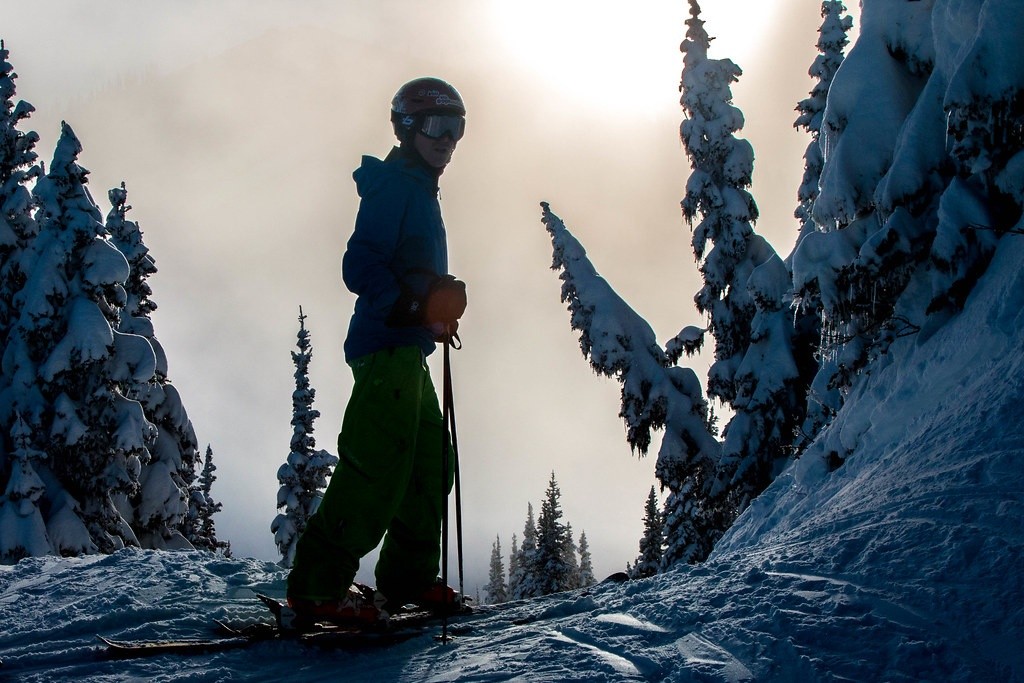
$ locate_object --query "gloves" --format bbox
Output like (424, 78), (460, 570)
(420, 273), (467, 342)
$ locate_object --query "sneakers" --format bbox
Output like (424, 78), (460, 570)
(286, 580), (388, 627)
(378, 575), (472, 614)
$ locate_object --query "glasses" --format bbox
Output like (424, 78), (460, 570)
(411, 115), (465, 142)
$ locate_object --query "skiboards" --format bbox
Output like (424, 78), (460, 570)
(93, 601), (475, 656)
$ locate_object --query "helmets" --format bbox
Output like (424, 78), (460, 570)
(391, 78), (466, 138)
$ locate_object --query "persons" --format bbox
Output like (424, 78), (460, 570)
(255, 77), (473, 630)
